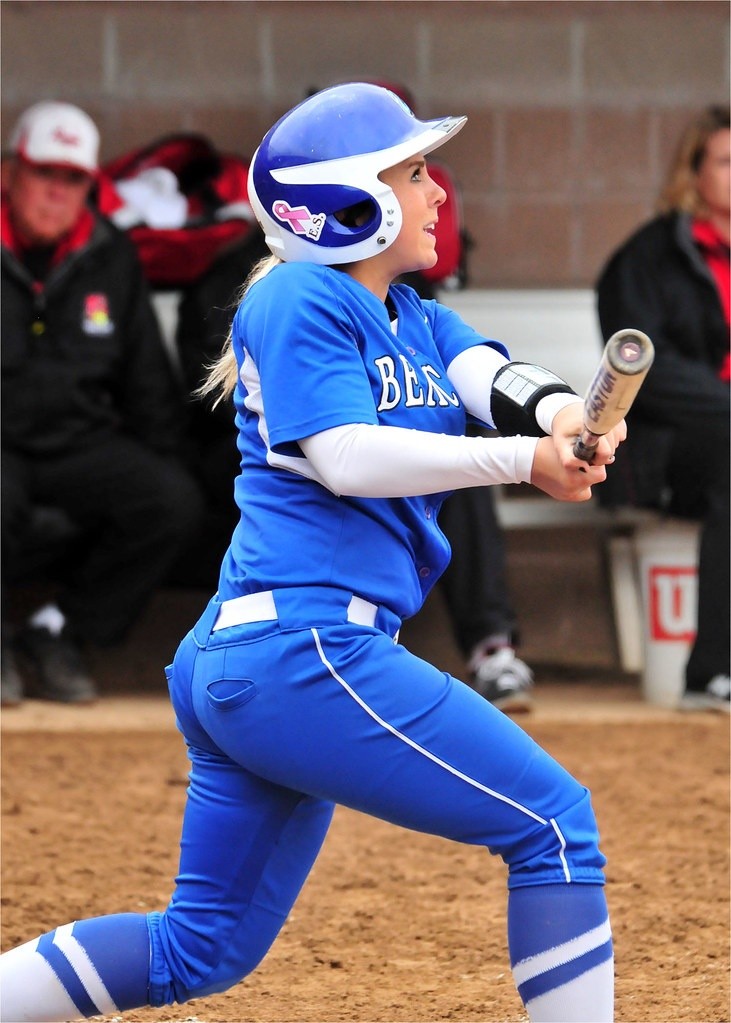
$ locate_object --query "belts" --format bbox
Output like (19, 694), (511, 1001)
(203, 586), (405, 645)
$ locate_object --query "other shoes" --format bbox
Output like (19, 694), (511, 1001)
(1, 622), (21, 706)
(23, 629), (98, 703)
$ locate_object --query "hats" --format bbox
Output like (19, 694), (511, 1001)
(246, 82), (469, 266)
(5, 98), (101, 179)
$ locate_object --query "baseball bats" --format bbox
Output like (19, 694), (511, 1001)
(571, 329), (655, 465)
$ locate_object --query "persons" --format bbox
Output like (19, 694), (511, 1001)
(0, 80), (632, 1023)
(179, 229), (543, 713)
(597, 100), (730, 713)
(0, 97), (204, 705)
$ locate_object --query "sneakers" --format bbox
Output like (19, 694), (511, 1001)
(683, 674), (730, 713)
(465, 646), (534, 713)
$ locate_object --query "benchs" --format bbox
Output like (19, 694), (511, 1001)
(151, 283), (670, 678)
